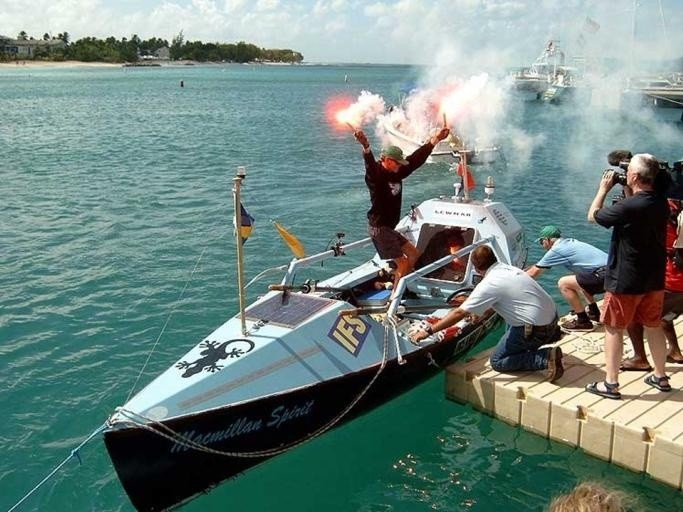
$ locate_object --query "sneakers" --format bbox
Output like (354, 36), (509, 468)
(546, 346), (564, 383)
(560, 305), (600, 332)
(386, 286), (420, 320)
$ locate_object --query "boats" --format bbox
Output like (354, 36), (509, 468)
(262, 58), (292, 66)
(381, 89), (500, 166)
(122, 62), (161, 68)
(97, 188), (533, 512)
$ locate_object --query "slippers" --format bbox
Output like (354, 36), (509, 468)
(666, 354), (683, 364)
(620, 357), (652, 371)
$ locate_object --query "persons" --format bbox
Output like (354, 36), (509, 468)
(616, 179), (683, 371)
(584, 152), (674, 399)
(521, 221), (612, 333)
(407, 243), (568, 383)
(344, 117), (454, 297)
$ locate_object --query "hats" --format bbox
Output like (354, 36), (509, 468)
(380, 145), (410, 166)
(533, 225), (562, 243)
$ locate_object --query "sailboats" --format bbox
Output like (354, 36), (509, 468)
(501, 0), (683, 108)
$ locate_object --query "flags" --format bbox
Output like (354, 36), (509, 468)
(232, 195), (256, 247)
(274, 223), (307, 260)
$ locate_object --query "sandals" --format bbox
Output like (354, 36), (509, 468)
(644, 375), (672, 392)
(584, 380), (621, 399)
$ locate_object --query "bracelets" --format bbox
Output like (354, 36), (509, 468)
(424, 326), (435, 336)
